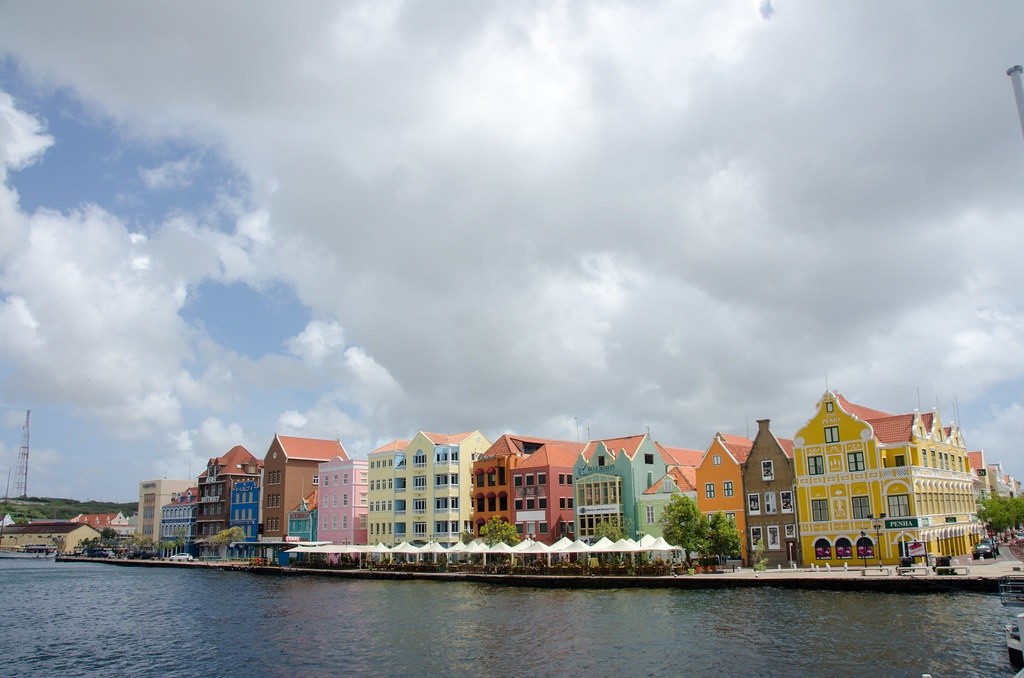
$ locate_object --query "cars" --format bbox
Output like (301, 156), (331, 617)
(981, 538), (999, 556)
(972, 543), (992, 560)
(169, 553), (193, 562)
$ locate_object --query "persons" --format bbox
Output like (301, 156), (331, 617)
(248, 552), (728, 576)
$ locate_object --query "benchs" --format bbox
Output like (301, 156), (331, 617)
(862, 568), (893, 576)
(897, 567), (930, 576)
(936, 566), (970, 575)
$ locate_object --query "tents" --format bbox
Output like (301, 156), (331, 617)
(285, 532), (681, 571)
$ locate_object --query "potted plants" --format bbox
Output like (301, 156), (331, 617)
(687, 566), (695, 575)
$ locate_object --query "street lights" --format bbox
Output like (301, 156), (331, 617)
(866, 513), (886, 572)
